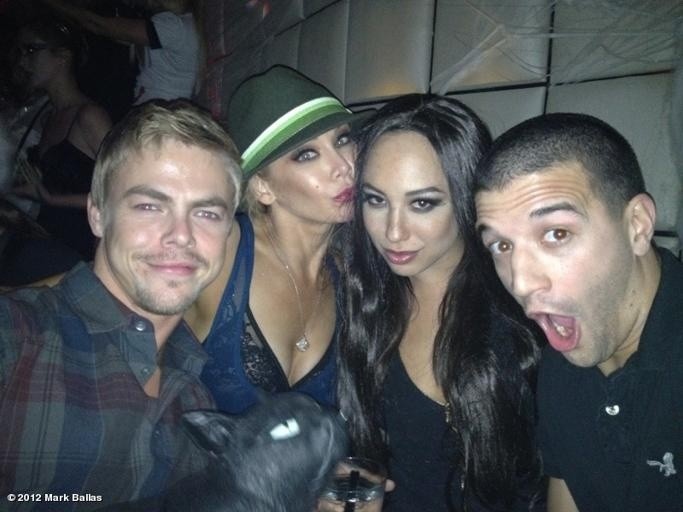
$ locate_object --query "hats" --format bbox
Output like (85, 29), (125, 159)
(224, 65), (378, 178)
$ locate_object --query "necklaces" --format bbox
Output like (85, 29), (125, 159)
(261, 212), (324, 351)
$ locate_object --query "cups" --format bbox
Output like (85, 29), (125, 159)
(313, 456), (387, 511)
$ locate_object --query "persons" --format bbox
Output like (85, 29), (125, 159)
(310, 91), (550, 510)
(1, 97), (243, 512)
(1, 12), (116, 289)
(472, 112), (682, 512)
(43, 0), (201, 128)
(1, 66), (357, 419)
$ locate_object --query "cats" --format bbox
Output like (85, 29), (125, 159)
(91, 388), (348, 512)
